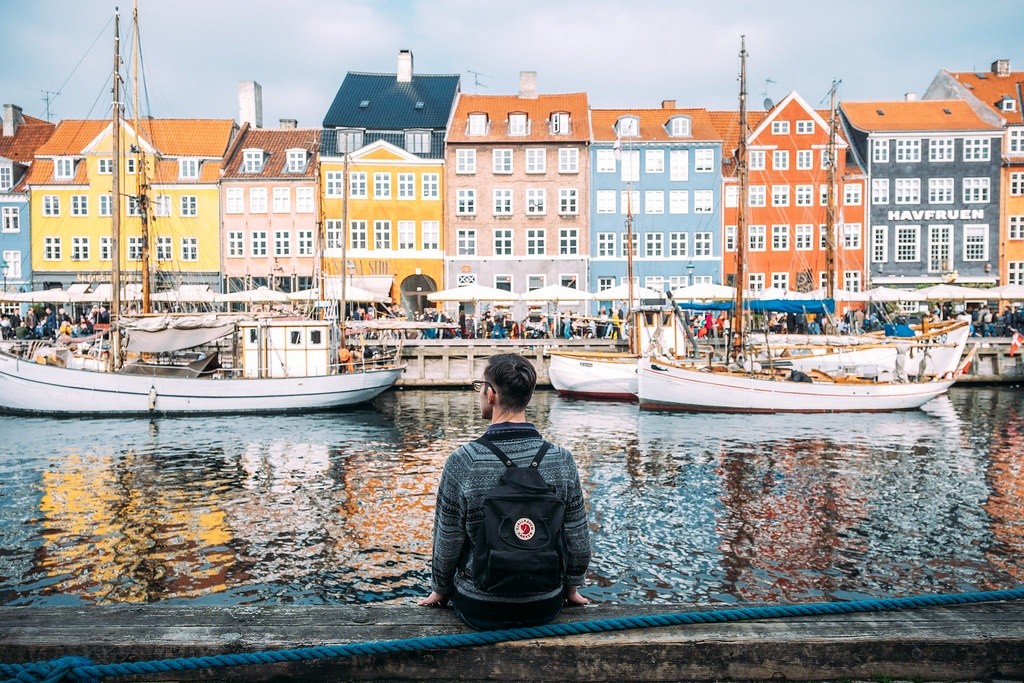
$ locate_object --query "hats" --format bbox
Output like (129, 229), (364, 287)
(792, 313), (797, 316)
(772, 311), (778, 314)
(784, 312), (788, 316)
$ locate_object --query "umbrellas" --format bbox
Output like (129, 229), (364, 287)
(427, 284), (1024, 313)
(0, 286), (392, 307)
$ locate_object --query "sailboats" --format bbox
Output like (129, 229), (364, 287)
(0, 0), (1023, 415)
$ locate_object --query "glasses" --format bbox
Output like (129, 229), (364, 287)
(473, 379), (496, 395)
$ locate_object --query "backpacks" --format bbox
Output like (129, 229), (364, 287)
(474, 438), (567, 597)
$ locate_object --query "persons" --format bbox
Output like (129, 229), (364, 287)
(417, 352), (589, 631)
(0, 305), (1024, 344)
